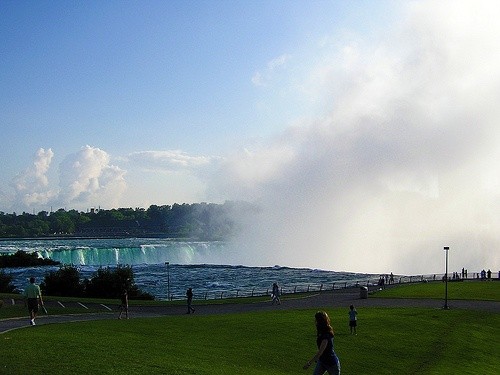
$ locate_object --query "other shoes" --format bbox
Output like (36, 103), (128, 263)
(118, 317), (121, 319)
(192, 309), (196, 313)
(354, 332), (357, 334)
(187, 311), (191, 313)
(31, 319), (36, 325)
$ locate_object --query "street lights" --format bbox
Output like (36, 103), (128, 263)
(443, 246), (450, 310)
(164, 262), (170, 301)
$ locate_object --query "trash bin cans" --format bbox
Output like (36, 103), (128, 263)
(360, 286), (368, 299)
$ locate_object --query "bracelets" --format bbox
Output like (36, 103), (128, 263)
(308, 360), (311, 365)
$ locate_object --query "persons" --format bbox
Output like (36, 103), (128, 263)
(271, 283), (281, 305)
(379, 269), (492, 289)
(186, 288), (196, 313)
(303, 310), (341, 375)
(118, 290), (129, 320)
(348, 304), (359, 335)
(23, 277), (44, 325)
